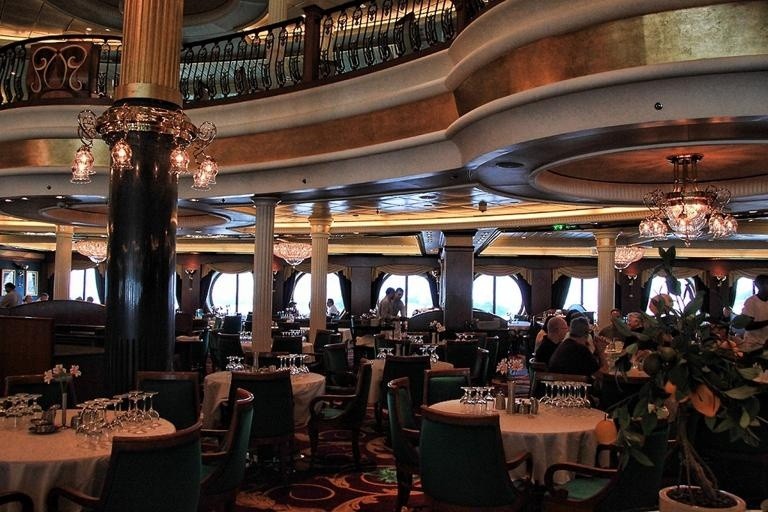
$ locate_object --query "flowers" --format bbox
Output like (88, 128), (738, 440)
(606, 245), (768, 498)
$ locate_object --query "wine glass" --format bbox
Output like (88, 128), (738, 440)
(225, 356), (245, 376)
(277, 353), (310, 377)
(76, 390), (159, 446)
(0, 392), (43, 430)
(377, 348), (394, 360)
(458, 385), (495, 413)
(281, 329), (307, 345)
(238, 331), (252, 343)
(408, 335), (423, 343)
(419, 344), (439, 365)
(539, 380), (592, 416)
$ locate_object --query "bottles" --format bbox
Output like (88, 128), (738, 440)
(496, 391), (538, 415)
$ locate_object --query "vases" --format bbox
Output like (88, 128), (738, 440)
(657, 482), (747, 512)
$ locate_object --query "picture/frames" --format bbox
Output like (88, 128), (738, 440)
(25, 271), (39, 297)
(1, 269), (16, 297)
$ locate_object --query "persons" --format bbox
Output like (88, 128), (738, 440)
(739, 274), (768, 390)
(2, 282), (50, 307)
(75, 295), (93, 304)
(376, 287), (406, 320)
(326, 298), (338, 318)
(533, 307), (654, 409)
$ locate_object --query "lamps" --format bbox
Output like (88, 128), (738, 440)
(639, 153), (739, 248)
(70, 103), (219, 191)
(614, 245), (646, 273)
(74, 239), (108, 267)
(273, 242), (312, 268)
